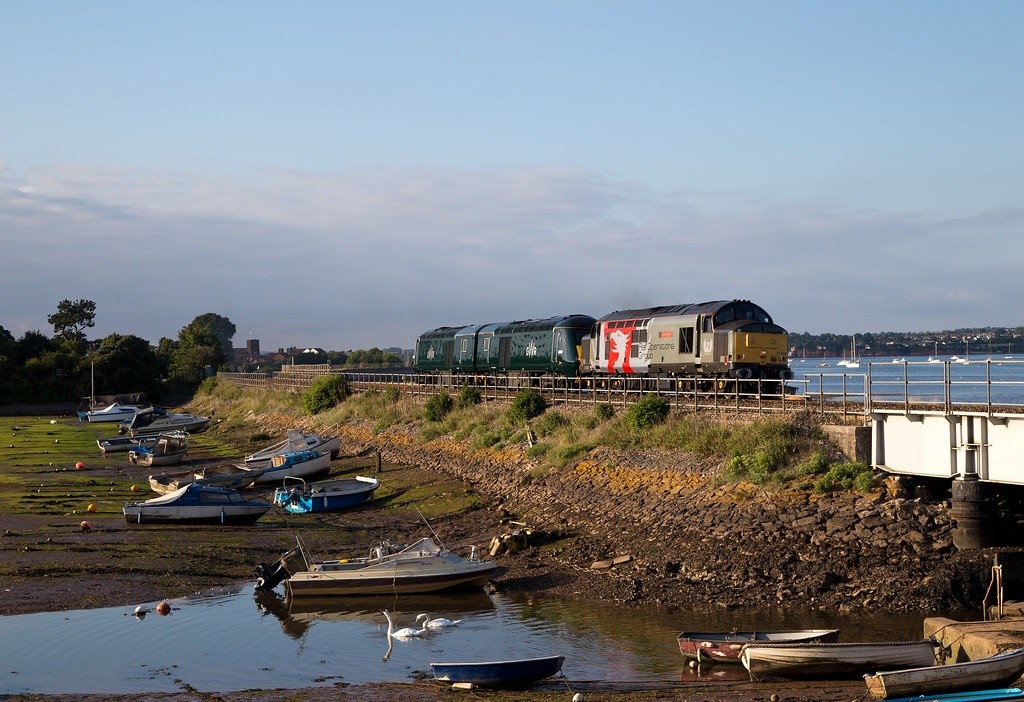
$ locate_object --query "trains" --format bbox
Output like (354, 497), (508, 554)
(413, 299), (795, 400)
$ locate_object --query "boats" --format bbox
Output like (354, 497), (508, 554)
(128, 434), (188, 466)
(837, 360), (850, 366)
(123, 482), (271, 524)
(254, 450), (331, 483)
(677, 628), (840, 662)
(862, 649), (1024, 702)
(87, 402), (140, 422)
(273, 476), (380, 514)
(951, 356), (959, 359)
(149, 464), (256, 496)
(96, 432), (190, 453)
(430, 655), (565, 684)
(251, 502), (509, 614)
(245, 423), (339, 461)
(119, 403), (211, 432)
(846, 362), (860, 368)
(738, 636), (941, 681)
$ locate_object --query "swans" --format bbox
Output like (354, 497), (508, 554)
(379, 608), (462, 637)
